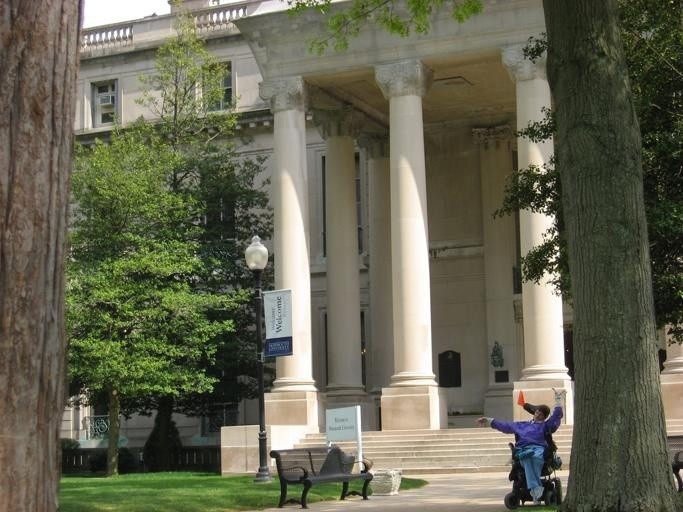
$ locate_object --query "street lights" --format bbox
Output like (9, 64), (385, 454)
(243, 236), (273, 485)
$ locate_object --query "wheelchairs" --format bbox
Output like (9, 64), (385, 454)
(503, 430), (564, 509)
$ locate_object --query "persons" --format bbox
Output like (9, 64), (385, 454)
(476, 386), (568, 506)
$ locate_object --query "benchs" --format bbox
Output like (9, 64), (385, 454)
(270, 447), (373, 509)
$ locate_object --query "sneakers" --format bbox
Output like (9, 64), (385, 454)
(533, 487), (544, 501)
(529, 490), (540, 506)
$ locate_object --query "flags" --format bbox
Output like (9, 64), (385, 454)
(261, 289), (294, 358)
